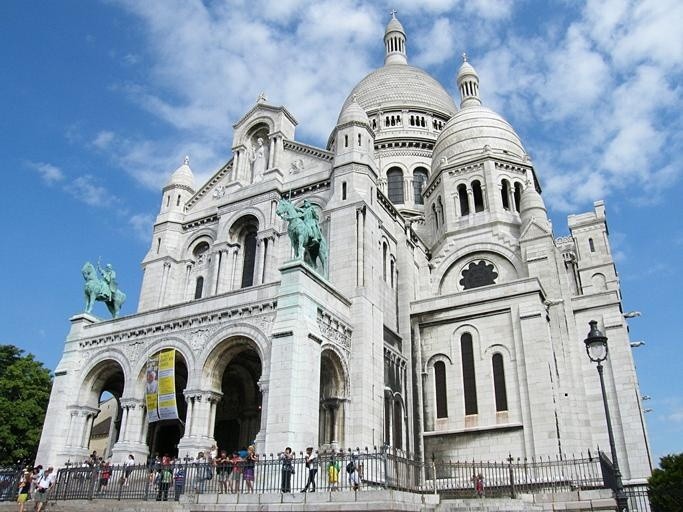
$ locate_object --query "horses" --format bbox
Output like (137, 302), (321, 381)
(276, 197), (328, 280)
(82, 262), (126, 319)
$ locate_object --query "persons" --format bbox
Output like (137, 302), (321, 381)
(95, 255), (116, 303)
(470, 471), (487, 499)
(298, 446), (318, 493)
(251, 136), (268, 177)
(345, 461), (362, 491)
(145, 369), (157, 393)
(294, 197), (321, 245)
(277, 447), (295, 493)
(325, 455), (341, 491)
(15, 461), (56, 512)
(83, 443), (259, 500)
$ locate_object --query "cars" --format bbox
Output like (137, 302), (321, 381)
(0, 467), (19, 502)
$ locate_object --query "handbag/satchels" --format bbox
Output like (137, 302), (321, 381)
(284, 464), (295, 474)
(345, 461), (356, 474)
(207, 466), (213, 480)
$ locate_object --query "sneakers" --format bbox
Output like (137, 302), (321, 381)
(352, 484), (358, 491)
(218, 490), (254, 494)
(281, 489), (314, 493)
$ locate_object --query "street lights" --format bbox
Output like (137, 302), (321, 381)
(582, 318), (630, 512)
